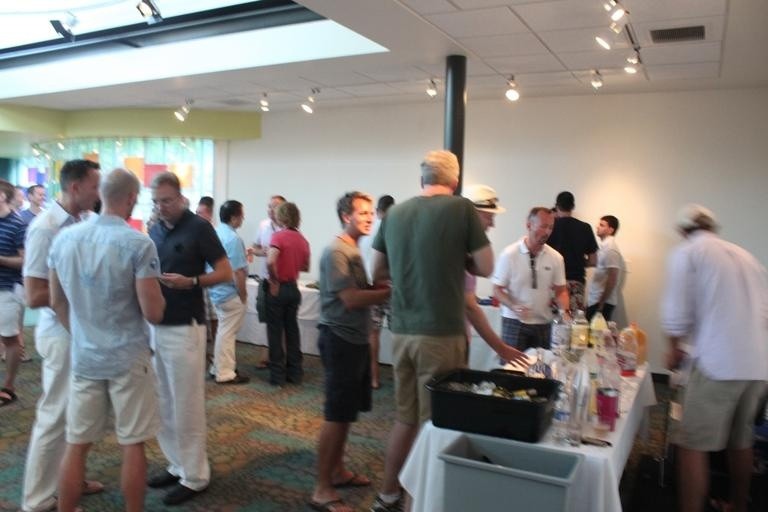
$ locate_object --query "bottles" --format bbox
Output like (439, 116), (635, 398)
(588, 312), (608, 353)
(571, 309), (588, 355)
(551, 392), (570, 447)
(549, 309), (570, 355)
(622, 322), (645, 366)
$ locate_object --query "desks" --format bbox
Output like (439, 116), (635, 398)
(399, 346), (656, 510)
(235, 277), (503, 368)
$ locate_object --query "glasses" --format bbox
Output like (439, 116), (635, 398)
(151, 195), (184, 206)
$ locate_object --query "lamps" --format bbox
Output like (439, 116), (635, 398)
(426, 79), (438, 98)
(260, 92), (270, 112)
(624, 52), (640, 75)
(596, 1), (629, 51)
(505, 76), (520, 102)
(590, 69), (603, 89)
(301, 86), (321, 114)
(174, 97), (193, 123)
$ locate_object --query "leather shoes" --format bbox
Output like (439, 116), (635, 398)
(147, 467), (180, 488)
(73, 480), (105, 496)
(161, 480), (196, 507)
(64, 505), (89, 512)
(216, 373), (251, 385)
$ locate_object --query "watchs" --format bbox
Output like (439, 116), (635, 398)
(192, 274), (202, 290)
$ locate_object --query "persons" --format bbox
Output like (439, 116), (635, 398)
(463, 185), (529, 371)
(492, 207), (569, 363)
(142, 210), (161, 233)
(373, 195), (404, 390)
(309, 191), (389, 512)
(248, 195), (288, 370)
(48, 168), (164, 512)
(197, 197), (219, 380)
(267, 203), (309, 387)
(370, 150), (493, 512)
(548, 191), (598, 312)
(206, 200), (253, 383)
(586, 216), (625, 322)
(145, 172), (231, 505)
(1, 181), (22, 411)
(21, 185), (43, 234)
(21, 161), (104, 511)
(663, 203), (768, 512)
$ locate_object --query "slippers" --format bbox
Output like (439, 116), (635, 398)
(305, 494), (354, 512)
(333, 472), (371, 488)
(253, 360), (271, 369)
(0, 384), (18, 406)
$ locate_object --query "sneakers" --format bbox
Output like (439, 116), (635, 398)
(368, 493), (405, 512)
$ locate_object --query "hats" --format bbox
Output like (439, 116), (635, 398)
(673, 201), (716, 233)
(472, 184), (508, 215)
